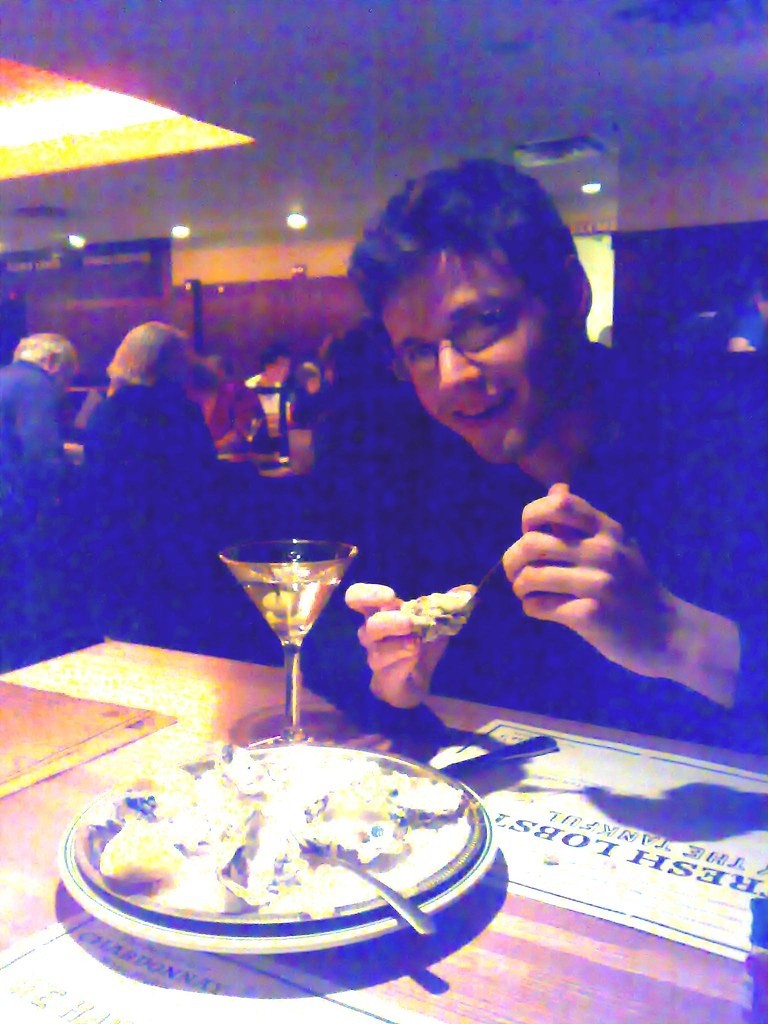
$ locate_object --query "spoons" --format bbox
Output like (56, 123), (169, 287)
(444, 554), (501, 615)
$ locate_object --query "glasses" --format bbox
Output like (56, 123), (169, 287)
(388, 301), (526, 382)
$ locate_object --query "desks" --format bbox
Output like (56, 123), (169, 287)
(0, 639), (768, 1024)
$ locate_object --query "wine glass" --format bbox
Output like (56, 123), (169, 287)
(217, 536), (359, 746)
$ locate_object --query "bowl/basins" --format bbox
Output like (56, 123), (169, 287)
(57, 745), (497, 952)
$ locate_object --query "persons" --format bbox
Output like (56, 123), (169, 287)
(302, 160), (768, 746)
(245, 345), (294, 450)
(0, 321), (242, 664)
(186, 360), (265, 454)
(726, 277), (768, 353)
(294, 363), (327, 427)
